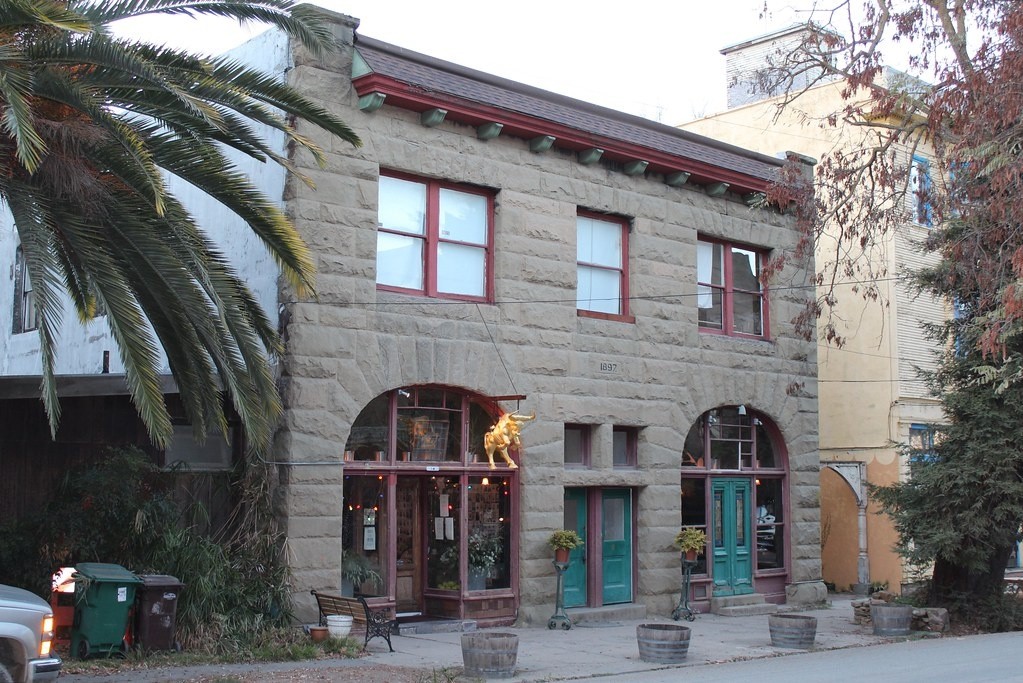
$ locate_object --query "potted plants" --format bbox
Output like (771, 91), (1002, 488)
(545, 529), (584, 562)
(340, 551), (388, 624)
(673, 525), (712, 561)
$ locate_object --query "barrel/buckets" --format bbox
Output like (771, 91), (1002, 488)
(460, 630), (519, 678)
(636, 623), (692, 664)
(870, 603), (912, 637)
(768, 614), (818, 649)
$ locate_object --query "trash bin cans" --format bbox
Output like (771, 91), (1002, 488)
(69, 562), (144, 661)
(132, 573), (186, 655)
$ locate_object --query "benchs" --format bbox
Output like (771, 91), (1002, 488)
(310, 590), (396, 653)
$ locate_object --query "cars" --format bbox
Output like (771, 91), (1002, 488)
(756, 511), (785, 553)
(0, 582), (63, 683)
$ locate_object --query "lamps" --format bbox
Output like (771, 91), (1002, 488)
(736, 404), (746, 415)
(396, 389), (410, 398)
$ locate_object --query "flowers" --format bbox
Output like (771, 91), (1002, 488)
(439, 531), (499, 578)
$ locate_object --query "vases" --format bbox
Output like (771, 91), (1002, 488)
(310, 615), (354, 641)
(468, 569), (485, 590)
